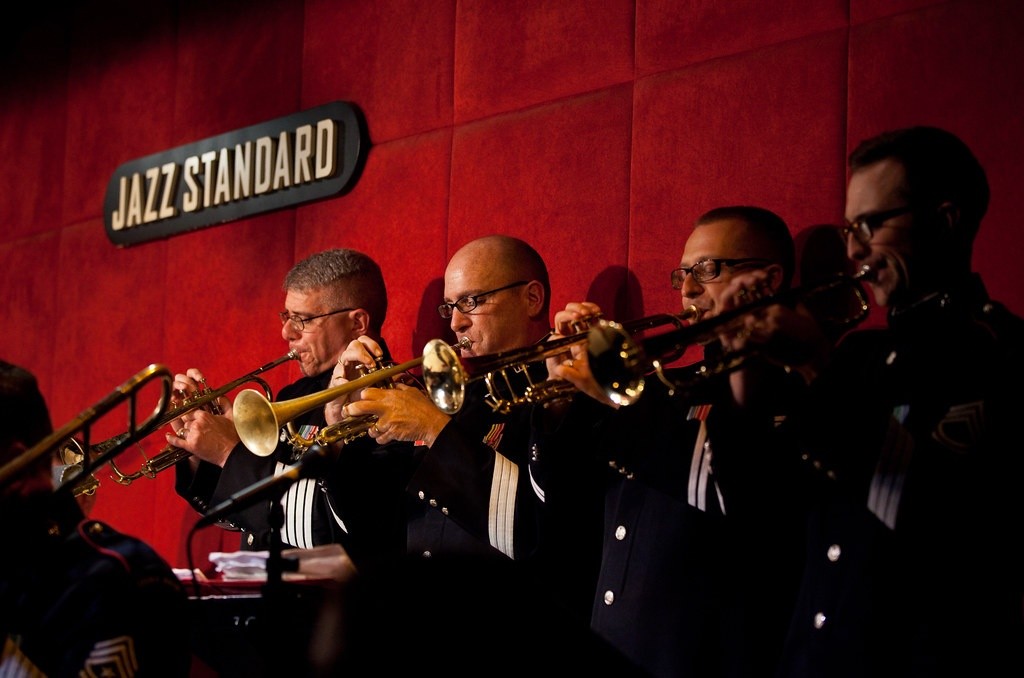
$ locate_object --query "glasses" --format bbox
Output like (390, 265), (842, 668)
(841, 205), (912, 243)
(437, 280), (528, 319)
(278, 307), (355, 331)
(672, 257), (779, 290)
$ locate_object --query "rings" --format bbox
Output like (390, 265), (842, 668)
(372, 424), (390, 436)
(176, 427), (189, 440)
(570, 358), (577, 367)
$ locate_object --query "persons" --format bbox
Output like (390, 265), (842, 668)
(155, 248), (409, 678)
(0, 359), (196, 677)
(716, 123), (1024, 677)
(324, 228), (601, 678)
(550, 204), (850, 677)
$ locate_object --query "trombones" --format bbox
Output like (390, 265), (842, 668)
(0, 358), (175, 518)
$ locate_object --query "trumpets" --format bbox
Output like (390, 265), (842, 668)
(231, 334), (473, 458)
(585, 253), (891, 408)
(421, 304), (703, 416)
(38, 347), (304, 501)
